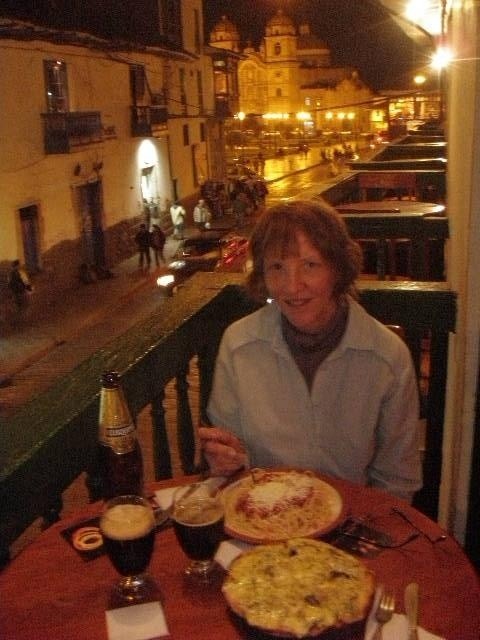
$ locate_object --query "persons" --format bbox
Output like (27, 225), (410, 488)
(7, 258), (35, 322)
(150, 224), (168, 263)
(277, 140), (356, 177)
(170, 199), (187, 240)
(96, 257), (120, 281)
(136, 224), (151, 266)
(198, 199), (423, 506)
(201, 150), (269, 229)
(193, 197), (211, 233)
(77, 263), (95, 283)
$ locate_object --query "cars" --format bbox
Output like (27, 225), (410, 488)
(159, 233), (249, 303)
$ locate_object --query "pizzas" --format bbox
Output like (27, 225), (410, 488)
(221, 539), (376, 638)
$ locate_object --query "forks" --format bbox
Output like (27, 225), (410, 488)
(372, 584), (397, 640)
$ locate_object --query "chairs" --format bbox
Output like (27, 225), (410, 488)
(333, 170), (447, 282)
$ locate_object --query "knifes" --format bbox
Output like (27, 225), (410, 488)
(404, 578), (424, 640)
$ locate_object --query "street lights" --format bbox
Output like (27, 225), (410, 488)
(430, 52), (449, 121)
(237, 112), (246, 162)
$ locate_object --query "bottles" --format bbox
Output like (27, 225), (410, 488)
(96, 371), (143, 513)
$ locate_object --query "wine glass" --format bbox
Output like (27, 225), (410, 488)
(98, 481), (226, 601)
